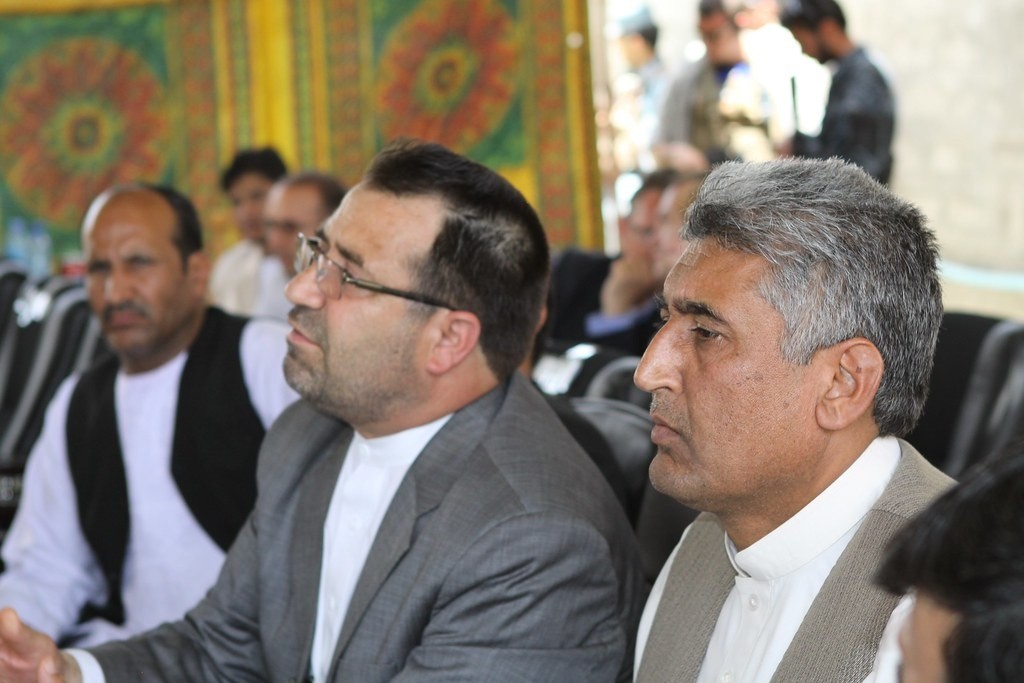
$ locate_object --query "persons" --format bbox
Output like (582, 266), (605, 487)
(794, 0), (898, 182)
(652, 0), (772, 169)
(0, 182), (301, 646)
(650, 173), (702, 262)
(544, 168), (679, 356)
(210, 145), (288, 316)
(612, 8), (664, 164)
(861, 443), (1024, 683)
(623, 158), (963, 683)
(252, 172), (345, 320)
(1, 136), (645, 683)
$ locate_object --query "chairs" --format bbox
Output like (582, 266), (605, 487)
(546, 311), (1024, 633)
(0, 263), (109, 577)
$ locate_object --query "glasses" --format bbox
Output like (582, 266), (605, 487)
(293, 230), (460, 314)
